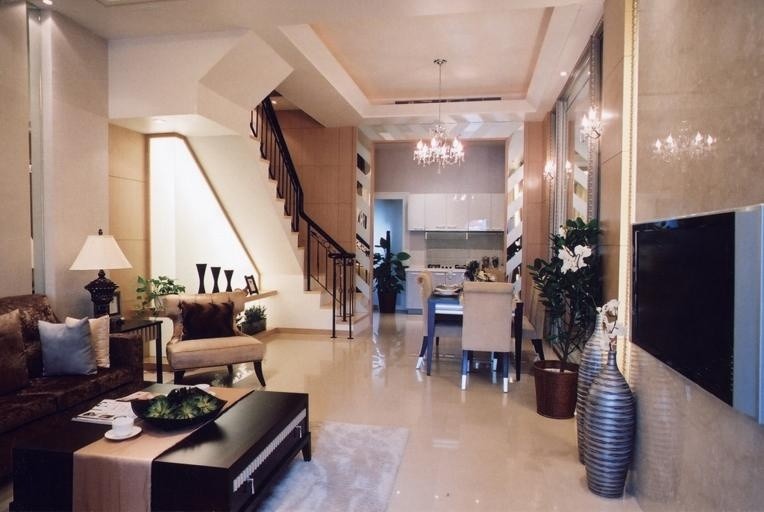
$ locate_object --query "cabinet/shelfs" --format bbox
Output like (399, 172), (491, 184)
(407, 192), (504, 234)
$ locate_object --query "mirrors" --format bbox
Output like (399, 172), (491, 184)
(550, 36), (601, 367)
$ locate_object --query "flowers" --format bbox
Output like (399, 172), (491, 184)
(527, 214), (606, 368)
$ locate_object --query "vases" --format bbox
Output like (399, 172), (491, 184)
(196, 264), (233, 293)
(531, 359), (577, 419)
(578, 313), (635, 498)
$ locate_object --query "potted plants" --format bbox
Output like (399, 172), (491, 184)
(372, 237), (411, 313)
(242, 305), (266, 335)
(136, 276), (185, 316)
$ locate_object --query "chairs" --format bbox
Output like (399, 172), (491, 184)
(161, 293), (268, 386)
(416, 272), (545, 393)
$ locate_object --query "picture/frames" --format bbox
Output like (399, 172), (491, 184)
(245, 274), (260, 295)
(108, 290), (121, 315)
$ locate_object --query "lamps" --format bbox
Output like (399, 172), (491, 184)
(68, 228), (131, 317)
(411, 60), (465, 176)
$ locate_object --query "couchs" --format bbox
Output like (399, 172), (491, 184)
(1, 294), (144, 490)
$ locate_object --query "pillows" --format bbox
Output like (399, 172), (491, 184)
(179, 301), (236, 340)
(0, 308), (31, 396)
(36, 314), (110, 378)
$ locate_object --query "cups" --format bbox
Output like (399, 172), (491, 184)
(111, 415), (134, 437)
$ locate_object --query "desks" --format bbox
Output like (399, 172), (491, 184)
(108, 318), (163, 383)
(126, 289), (278, 317)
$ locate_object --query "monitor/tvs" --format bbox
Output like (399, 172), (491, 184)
(628, 201), (763, 424)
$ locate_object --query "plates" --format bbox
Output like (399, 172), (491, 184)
(434, 292), (458, 298)
(103, 427), (143, 441)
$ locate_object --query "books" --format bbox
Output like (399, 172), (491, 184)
(70, 398), (143, 425)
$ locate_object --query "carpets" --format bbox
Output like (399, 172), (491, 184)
(254, 425), (411, 512)
